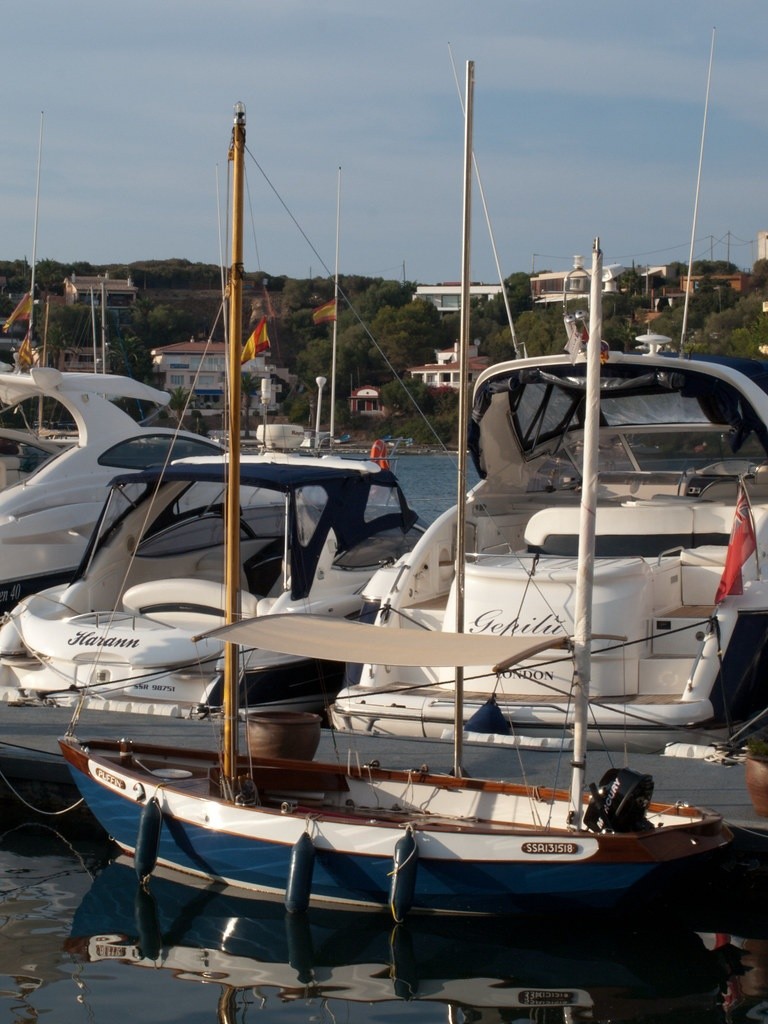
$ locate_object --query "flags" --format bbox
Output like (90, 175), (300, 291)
(714, 475), (757, 605)
(311, 298), (337, 324)
(2, 290), (33, 371)
(241, 316), (273, 363)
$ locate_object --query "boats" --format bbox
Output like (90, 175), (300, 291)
(0, 110), (430, 829)
(57, 26), (768, 1024)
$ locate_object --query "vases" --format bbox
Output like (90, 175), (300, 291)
(245, 712), (323, 761)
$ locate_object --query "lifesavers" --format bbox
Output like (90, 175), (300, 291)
(368, 439), (390, 472)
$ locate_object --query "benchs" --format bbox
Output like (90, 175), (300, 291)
(524, 496), (768, 556)
(123, 578), (257, 626)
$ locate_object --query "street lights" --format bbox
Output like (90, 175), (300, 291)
(315, 376), (327, 451)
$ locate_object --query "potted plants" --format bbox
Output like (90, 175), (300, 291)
(746, 735), (768, 817)
